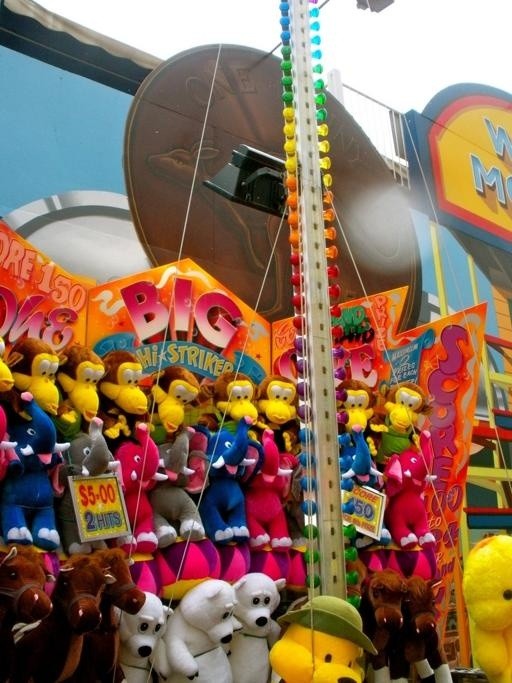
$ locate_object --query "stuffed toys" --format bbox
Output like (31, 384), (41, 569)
(0, 336), (452, 683)
(462, 534), (510, 681)
